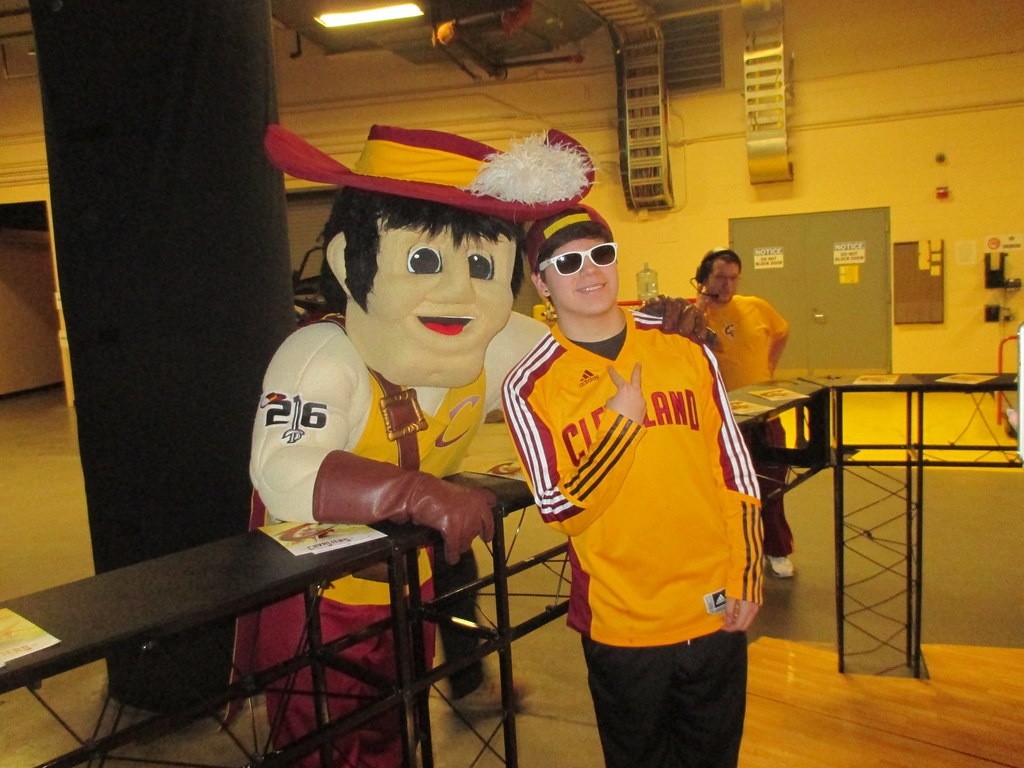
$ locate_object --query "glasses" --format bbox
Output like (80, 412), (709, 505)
(538, 242), (619, 276)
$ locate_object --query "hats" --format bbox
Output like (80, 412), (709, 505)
(525, 204), (613, 272)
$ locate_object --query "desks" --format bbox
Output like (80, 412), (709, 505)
(1, 370), (1023, 768)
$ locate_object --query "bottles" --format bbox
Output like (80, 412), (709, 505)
(636, 263), (659, 300)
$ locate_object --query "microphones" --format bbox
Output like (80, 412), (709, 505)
(690, 278), (719, 298)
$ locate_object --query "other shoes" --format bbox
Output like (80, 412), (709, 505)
(764, 553), (796, 577)
(450, 677), (524, 713)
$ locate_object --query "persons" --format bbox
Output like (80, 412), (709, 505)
(696, 250), (793, 579)
(500, 204), (764, 768)
(218, 125), (716, 768)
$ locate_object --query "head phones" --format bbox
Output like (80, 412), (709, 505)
(696, 247), (742, 285)
